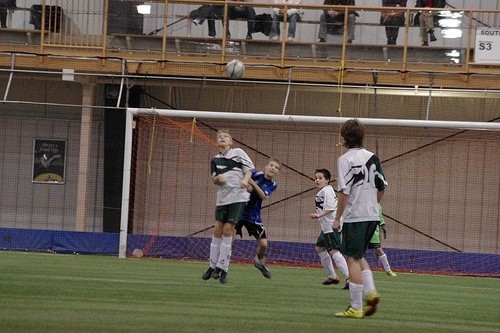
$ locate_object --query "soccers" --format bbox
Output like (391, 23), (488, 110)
(224, 59), (246, 79)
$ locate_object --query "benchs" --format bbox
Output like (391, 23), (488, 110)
(1, 7), (51, 43)
(114, 14), (477, 65)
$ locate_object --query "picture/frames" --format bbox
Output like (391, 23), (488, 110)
(31, 138), (67, 185)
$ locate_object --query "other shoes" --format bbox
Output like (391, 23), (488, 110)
(287, 36), (293, 42)
(387, 39), (396, 45)
(422, 42), (429, 46)
(246, 35), (252, 41)
(386, 270), (397, 277)
(272, 36), (279, 42)
(430, 32), (437, 41)
(319, 39), (326, 42)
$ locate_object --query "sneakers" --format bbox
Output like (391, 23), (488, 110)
(343, 282), (349, 289)
(322, 275), (340, 285)
(220, 270), (228, 284)
(363, 291), (380, 316)
(202, 267), (214, 280)
(252, 258), (272, 279)
(334, 306), (364, 319)
(211, 267), (222, 279)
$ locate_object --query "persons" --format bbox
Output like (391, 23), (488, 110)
(201, 4), (256, 39)
(0, 0), (16, 28)
(367, 202), (396, 278)
(310, 169), (349, 289)
(413, 0), (445, 46)
(380, 0), (407, 45)
(212, 157), (280, 278)
(269, 7), (304, 42)
(332, 118), (388, 318)
(202, 132), (256, 284)
(317, 0), (356, 44)
